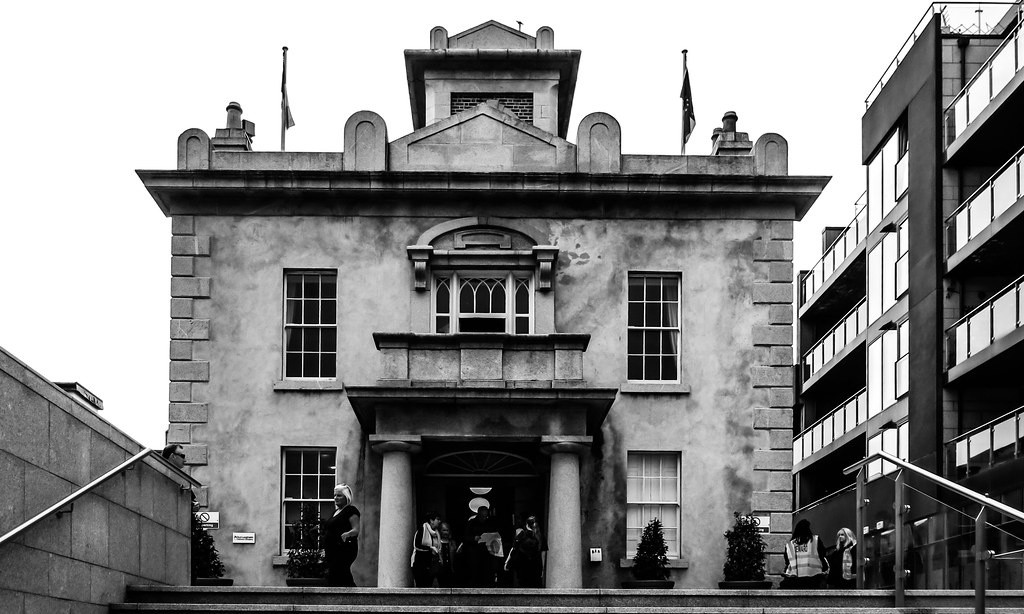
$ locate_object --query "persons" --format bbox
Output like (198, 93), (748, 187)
(829, 526), (871, 590)
(316, 483), (361, 587)
(408, 506), (549, 587)
(780, 519), (829, 589)
(880, 522), (923, 589)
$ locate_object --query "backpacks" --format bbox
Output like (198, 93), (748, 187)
(522, 540), (539, 568)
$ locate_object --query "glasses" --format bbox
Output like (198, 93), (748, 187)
(337, 482), (346, 486)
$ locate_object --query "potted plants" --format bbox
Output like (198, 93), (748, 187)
(718, 511), (773, 590)
(191, 511), (234, 586)
(281, 501), (328, 587)
(621, 517), (675, 589)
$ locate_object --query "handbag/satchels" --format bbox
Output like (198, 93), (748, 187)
(432, 553), (449, 575)
